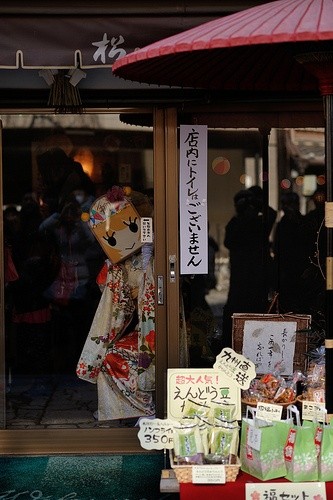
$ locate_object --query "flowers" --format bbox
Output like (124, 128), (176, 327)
(106, 185), (124, 202)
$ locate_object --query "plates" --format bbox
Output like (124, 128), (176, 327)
(241, 398), (297, 405)
(296, 395), (304, 403)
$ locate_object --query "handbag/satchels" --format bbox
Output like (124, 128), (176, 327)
(303, 405), (332, 482)
(273, 404), (319, 482)
(240, 406), (287, 481)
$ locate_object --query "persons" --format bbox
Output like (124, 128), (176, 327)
(3, 142), (153, 386)
(224, 185), (314, 344)
(76, 185), (188, 423)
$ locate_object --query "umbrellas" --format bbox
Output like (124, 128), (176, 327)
(113, 0), (333, 425)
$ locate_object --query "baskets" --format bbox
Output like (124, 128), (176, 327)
(169, 449), (242, 484)
